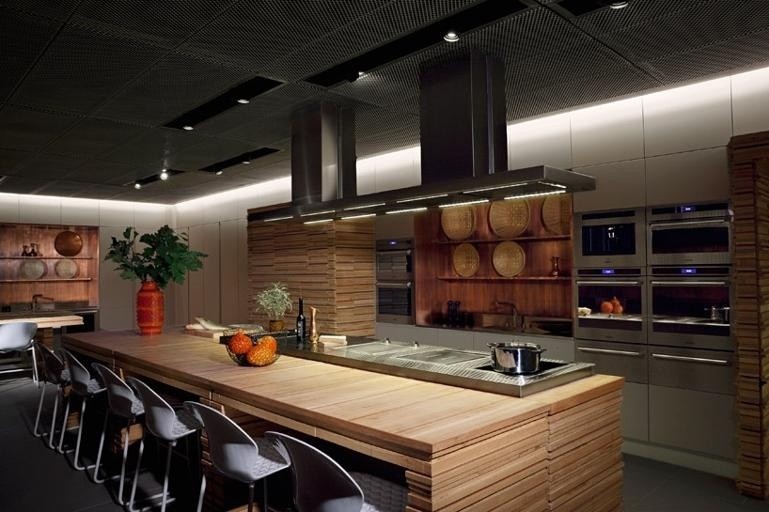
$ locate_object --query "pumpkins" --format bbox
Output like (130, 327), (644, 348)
(601, 296), (623, 313)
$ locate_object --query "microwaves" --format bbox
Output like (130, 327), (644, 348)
(374, 237), (415, 324)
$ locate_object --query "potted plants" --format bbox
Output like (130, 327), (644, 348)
(253, 281), (295, 331)
(104, 225), (211, 336)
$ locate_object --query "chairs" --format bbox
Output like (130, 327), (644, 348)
(91, 361), (193, 507)
(267, 431), (377, 512)
(182, 402), (294, 511)
(124, 375), (202, 511)
(57, 347), (130, 471)
(0, 322), (40, 388)
(34, 344), (98, 450)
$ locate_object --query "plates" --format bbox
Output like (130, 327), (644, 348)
(19, 259), (79, 280)
(453, 242), (527, 278)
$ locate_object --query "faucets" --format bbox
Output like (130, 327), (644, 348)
(31, 293), (54, 312)
(493, 299), (517, 323)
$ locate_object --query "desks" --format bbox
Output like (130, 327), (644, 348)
(0, 310), (85, 381)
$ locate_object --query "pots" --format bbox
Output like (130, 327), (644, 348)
(486, 341), (547, 374)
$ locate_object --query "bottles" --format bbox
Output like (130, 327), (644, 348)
(551, 256), (561, 277)
(21, 243), (38, 256)
(295, 297), (319, 344)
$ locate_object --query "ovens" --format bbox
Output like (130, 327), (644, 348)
(573, 205), (738, 396)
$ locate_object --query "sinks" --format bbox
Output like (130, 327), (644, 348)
(486, 324), (515, 335)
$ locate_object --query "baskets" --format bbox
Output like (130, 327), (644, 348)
(222, 329), (287, 366)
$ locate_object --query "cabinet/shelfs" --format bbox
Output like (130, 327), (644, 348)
(1, 222), (100, 315)
(413, 189), (575, 338)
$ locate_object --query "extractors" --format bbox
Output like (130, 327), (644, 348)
(247, 45), (597, 226)
(380, 49), (595, 216)
(248, 96), (385, 225)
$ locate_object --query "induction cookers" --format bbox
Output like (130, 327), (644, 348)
(475, 360), (569, 378)
